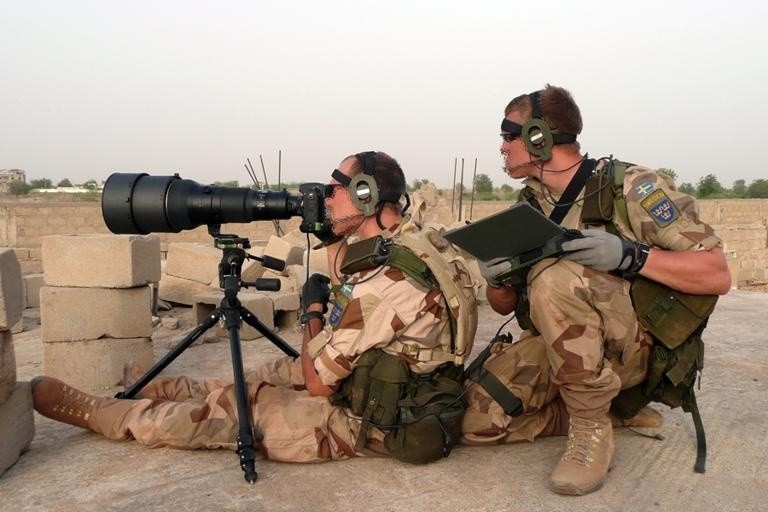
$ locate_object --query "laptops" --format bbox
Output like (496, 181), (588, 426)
(442, 200), (586, 288)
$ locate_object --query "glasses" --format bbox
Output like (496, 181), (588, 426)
(326, 184), (344, 196)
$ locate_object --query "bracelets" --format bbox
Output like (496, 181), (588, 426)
(632, 241), (650, 272)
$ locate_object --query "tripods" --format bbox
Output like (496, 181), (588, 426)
(113, 248), (301, 484)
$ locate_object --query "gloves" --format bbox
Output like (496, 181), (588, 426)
(561, 228), (638, 274)
(302, 273), (331, 314)
(476, 256), (515, 290)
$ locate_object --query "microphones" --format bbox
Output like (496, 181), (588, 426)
(331, 213), (364, 225)
(504, 157), (541, 175)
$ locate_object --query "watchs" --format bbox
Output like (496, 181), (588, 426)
(300, 311), (326, 332)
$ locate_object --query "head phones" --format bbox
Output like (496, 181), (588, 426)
(521, 91), (554, 162)
(349, 151), (380, 218)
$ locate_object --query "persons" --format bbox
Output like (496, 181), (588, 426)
(456, 86), (730, 495)
(30, 151), (478, 464)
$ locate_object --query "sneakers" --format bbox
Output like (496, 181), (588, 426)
(122, 358), (150, 400)
(549, 414), (619, 498)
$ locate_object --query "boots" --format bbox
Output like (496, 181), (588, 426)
(554, 395), (664, 438)
(31, 375), (99, 435)
